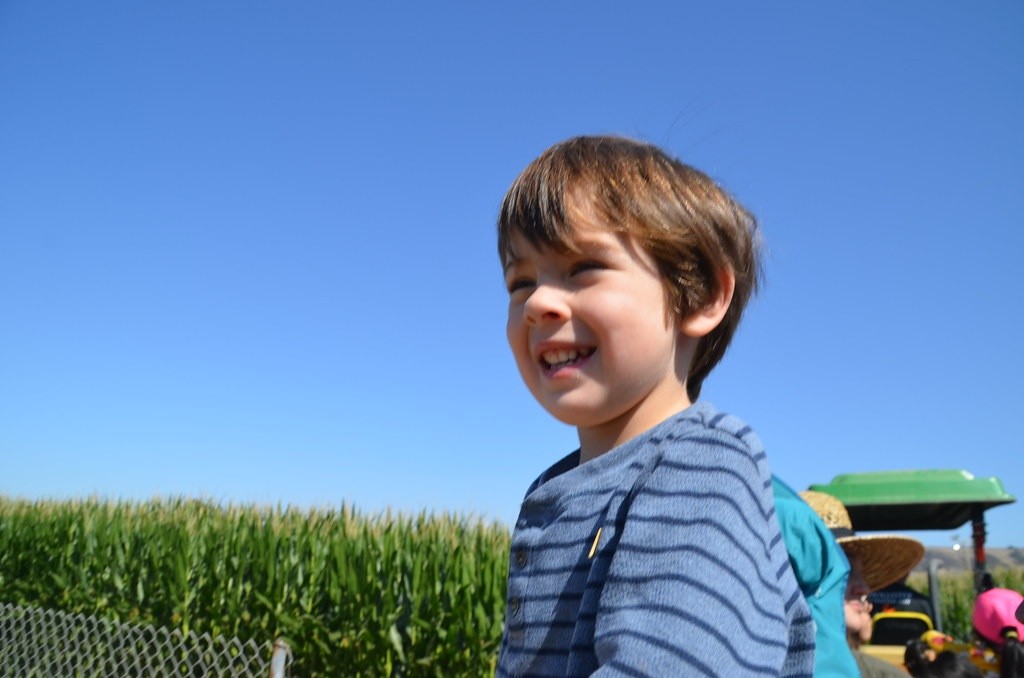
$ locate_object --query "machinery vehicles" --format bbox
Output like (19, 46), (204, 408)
(804, 468), (1019, 672)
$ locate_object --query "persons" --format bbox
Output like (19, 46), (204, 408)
(798, 491), (1024, 678)
(493, 134), (815, 678)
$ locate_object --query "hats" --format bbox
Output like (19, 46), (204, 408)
(971, 586), (1024, 649)
(796, 492), (923, 602)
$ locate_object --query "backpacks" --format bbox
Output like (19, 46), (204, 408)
(766, 474), (866, 678)
